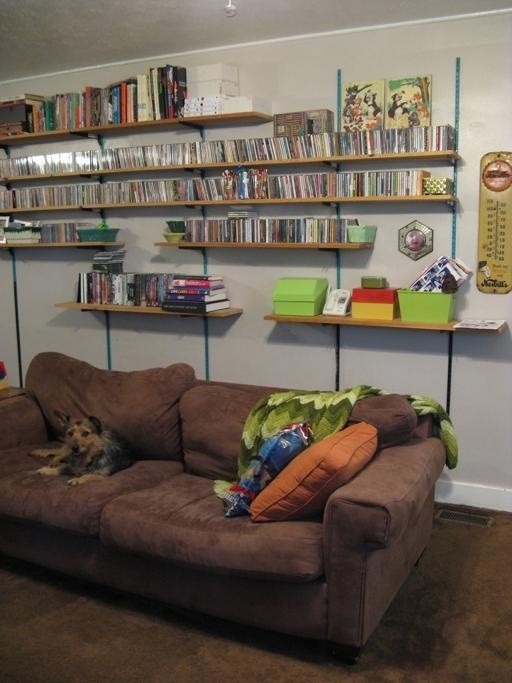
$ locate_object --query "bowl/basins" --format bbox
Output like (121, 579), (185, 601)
(76, 227), (120, 241)
(162, 231), (187, 241)
(165, 219), (186, 232)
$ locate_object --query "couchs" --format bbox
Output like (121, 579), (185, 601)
(0, 348), (446, 663)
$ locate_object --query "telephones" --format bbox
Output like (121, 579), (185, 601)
(322, 284), (351, 316)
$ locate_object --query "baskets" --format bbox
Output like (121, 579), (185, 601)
(347, 224), (377, 243)
(76, 228), (120, 242)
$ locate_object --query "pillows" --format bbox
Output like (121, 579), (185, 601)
(220, 420), (379, 524)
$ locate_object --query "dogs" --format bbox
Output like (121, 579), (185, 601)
(28, 407), (132, 487)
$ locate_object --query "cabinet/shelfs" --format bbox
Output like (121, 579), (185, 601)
(1, 108), (506, 334)
